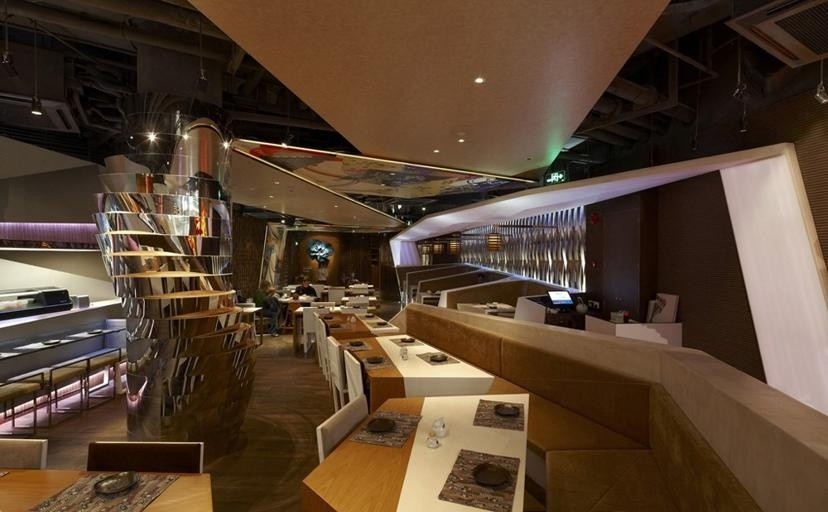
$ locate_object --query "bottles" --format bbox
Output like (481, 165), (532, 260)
(424, 417), (447, 449)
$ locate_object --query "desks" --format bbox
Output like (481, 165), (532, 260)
(1, 468), (214, 512)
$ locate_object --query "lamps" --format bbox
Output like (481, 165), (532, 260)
(421, 232), (464, 257)
(483, 221), (505, 253)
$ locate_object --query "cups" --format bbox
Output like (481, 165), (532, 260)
(609, 312), (625, 323)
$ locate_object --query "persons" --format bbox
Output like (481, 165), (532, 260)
(296, 276), (316, 306)
(256, 281), (280, 336)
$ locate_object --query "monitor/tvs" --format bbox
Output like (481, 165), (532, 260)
(547, 290), (574, 305)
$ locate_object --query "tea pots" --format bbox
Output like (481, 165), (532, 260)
(576, 296), (589, 314)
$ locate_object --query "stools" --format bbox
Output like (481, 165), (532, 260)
(0, 324), (126, 438)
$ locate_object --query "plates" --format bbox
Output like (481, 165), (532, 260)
(427, 288), (441, 296)
(366, 417), (396, 433)
(87, 328), (103, 334)
(473, 461), (509, 487)
(493, 403), (521, 417)
(40, 338), (61, 344)
(93, 471), (140, 494)
(317, 303), (450, 366)
(481, 300), (498, 310)
